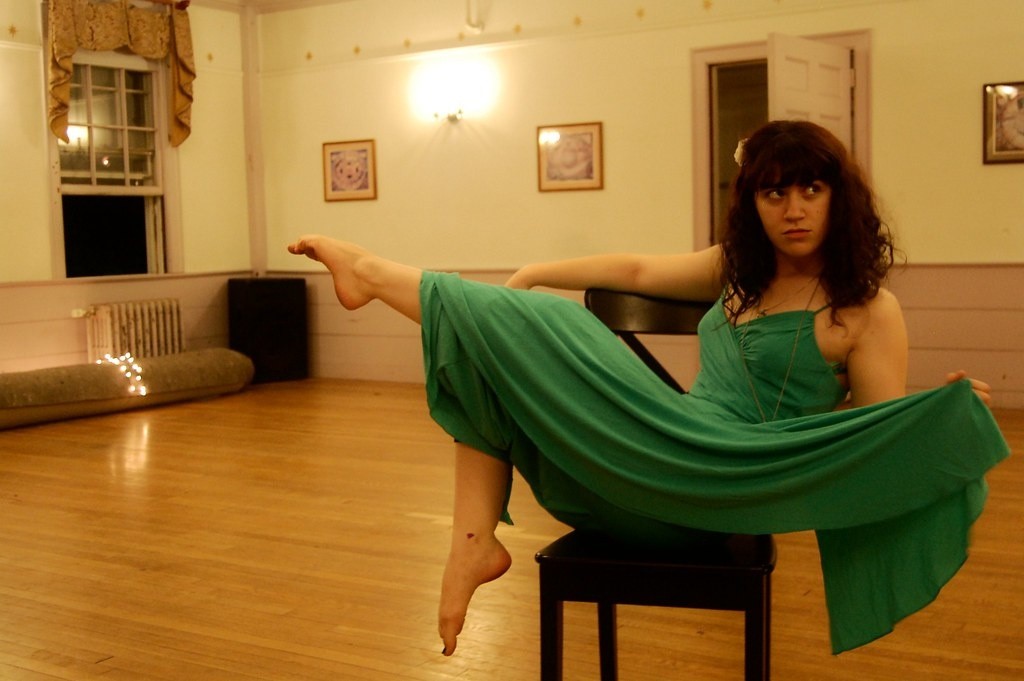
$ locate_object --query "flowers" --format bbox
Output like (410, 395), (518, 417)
(733, 137), (751, 167)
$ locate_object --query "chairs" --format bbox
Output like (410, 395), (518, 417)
(534, 288), (783, 681)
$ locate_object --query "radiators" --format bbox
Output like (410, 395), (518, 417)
(85, 301), (188, 364)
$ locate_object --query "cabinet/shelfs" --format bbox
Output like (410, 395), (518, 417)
(226, 277), (310, 383)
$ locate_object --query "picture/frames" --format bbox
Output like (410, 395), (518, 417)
(535, 119), (603, 192)
(982, 80), (1024, 165)
(321, 140), (375, 203)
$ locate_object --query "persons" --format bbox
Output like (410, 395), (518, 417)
(287, 120), (1014, 656)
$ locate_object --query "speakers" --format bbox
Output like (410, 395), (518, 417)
(227, 278), (309, 384)
